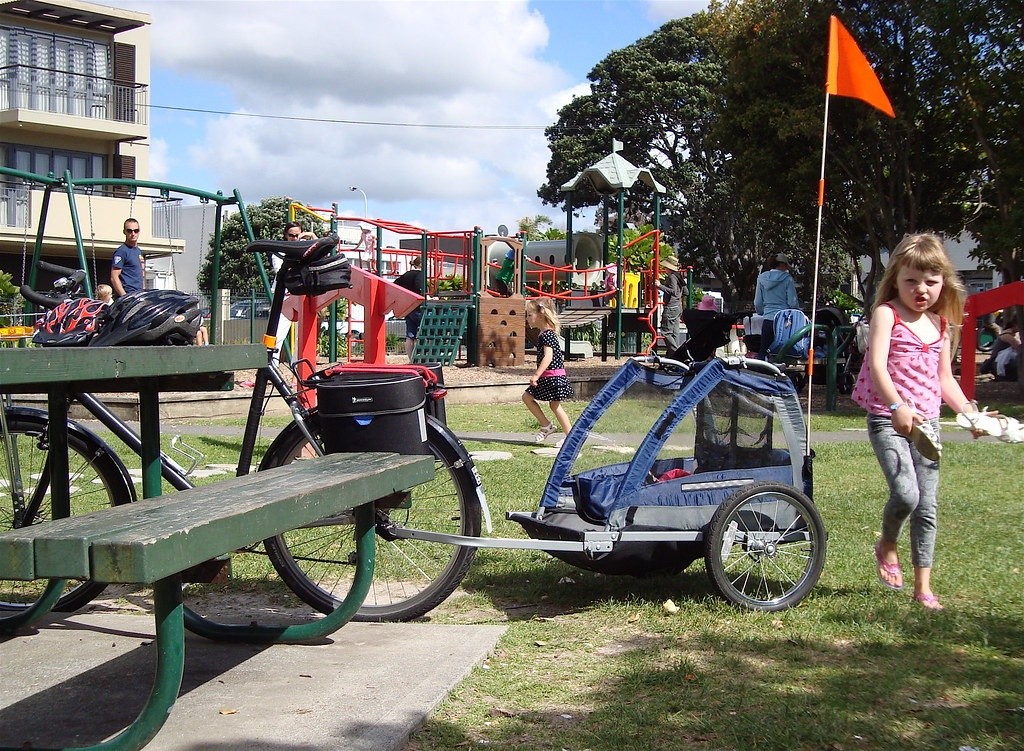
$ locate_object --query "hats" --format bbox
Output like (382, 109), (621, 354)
(605, 262), (617, 274)
(505, 249), (515, 261)
(775, 254), (792, 267)
(358, 222), (372, 231)
(660, 256), (679, 271)
(698, 296), (718, 310)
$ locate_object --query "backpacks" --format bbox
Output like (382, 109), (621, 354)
(767, 308), (811, 357)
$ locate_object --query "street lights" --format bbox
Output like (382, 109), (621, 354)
(348, 185), (369, 221)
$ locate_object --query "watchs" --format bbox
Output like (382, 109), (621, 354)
(888, 402), (908, 412)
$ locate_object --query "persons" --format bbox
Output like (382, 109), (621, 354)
(496, 249), (514, 290)
(522, 296), (573, 444)
(982, 312), (1021, 382)
(111, 218), (146, 298)
(754, 251), (799, 361)
(600, 263), (621, 306)
(196, 316), (209, 346)
(851, 234), (998, 612)
(393, 255), (423, 363)
(654, 256), (720, 359)
(353, 221), (377, 272)
(95, 284), (114, 306)
(270, 223), (318, 370)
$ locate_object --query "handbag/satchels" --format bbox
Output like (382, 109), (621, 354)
(980, 359), (991, 375)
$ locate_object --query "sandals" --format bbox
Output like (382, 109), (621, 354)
(876, 541), (903, 589)
(553, 438), (565, 448)
(535, 423), (557, 442)
(911, 412), (942, 462)
(955, 398), (1024, 443)
(913, 593), (938, 609)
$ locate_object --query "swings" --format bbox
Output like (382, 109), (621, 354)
(84, 185), (135, 301)
(1, 181), (33, 341)
(160, 188), (209, 339)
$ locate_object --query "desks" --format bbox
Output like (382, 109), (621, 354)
(0, 343), (377, 751)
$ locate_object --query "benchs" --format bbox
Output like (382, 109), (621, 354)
(0, 451), (434, 583)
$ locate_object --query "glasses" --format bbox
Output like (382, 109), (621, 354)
(287, 233), (298, 238)
(125, 229), (139, 234)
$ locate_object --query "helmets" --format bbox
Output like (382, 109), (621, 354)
(32, 298), (111, 344)
(89, 288), (204, 346)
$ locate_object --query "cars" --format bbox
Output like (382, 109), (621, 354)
(319, 309), (364, 348)
(199, 296), (271, 320)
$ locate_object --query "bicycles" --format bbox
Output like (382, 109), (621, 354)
(0, 232), (494, 637)
